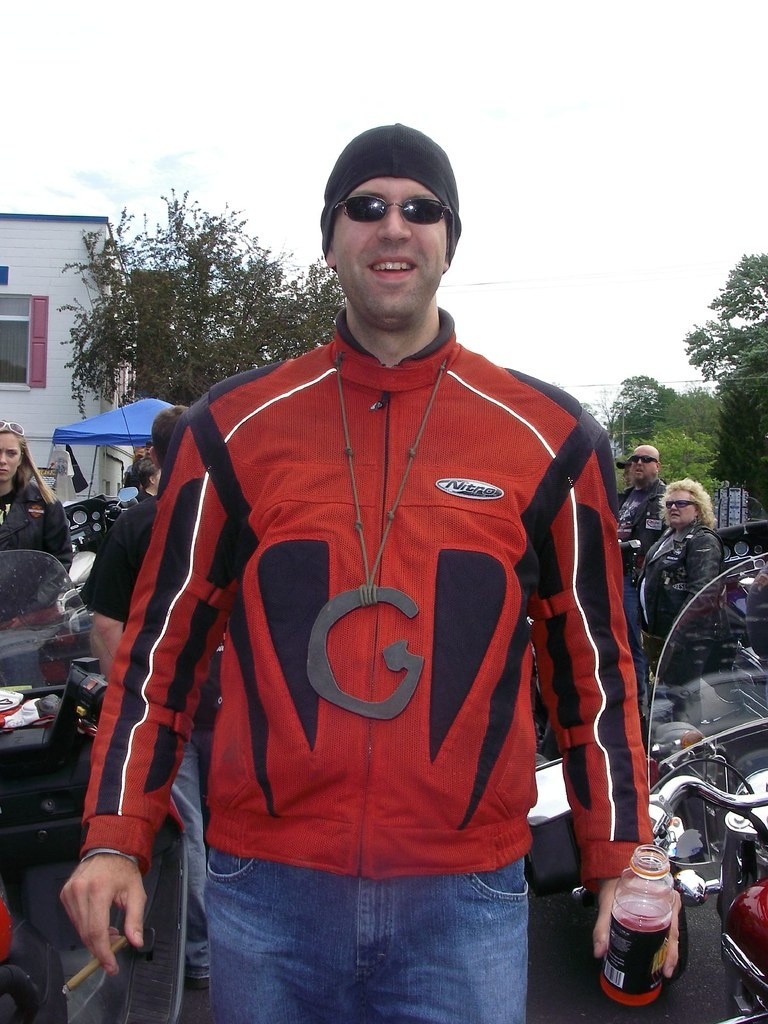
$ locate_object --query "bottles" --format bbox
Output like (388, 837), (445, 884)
(601, 845), (676, 1005)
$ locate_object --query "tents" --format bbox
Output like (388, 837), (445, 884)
(45, 398), (175, 495)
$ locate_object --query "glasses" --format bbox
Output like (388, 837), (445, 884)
(631, 455), (659, 463)
(0, 420), (25, 437)
(334, 195), (448, 225)
(665, 500), (696, 509)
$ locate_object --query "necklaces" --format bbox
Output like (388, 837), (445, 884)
(306, 354), (448, 719)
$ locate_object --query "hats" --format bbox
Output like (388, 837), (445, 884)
(320, 123), (462, 274)
(616, 455), (632, 469)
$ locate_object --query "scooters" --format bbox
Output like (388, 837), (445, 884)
(0, 488), (190, 1024)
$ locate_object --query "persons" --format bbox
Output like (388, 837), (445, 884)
(60, 123), (678, 1024)
(616, 442), (720, 686)
(0, 403), (209, 1024)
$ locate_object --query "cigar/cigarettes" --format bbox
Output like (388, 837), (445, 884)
(63, 936), (127, 994)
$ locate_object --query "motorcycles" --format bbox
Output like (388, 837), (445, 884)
(526, 519), (768, 1023)
